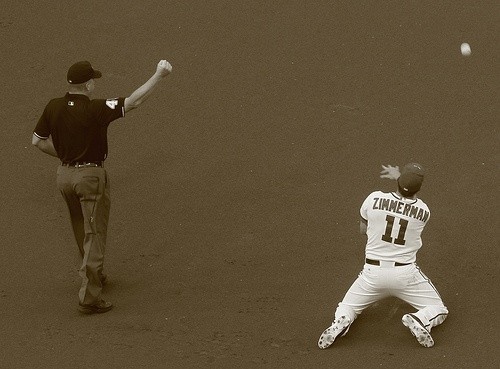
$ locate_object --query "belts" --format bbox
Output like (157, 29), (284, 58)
(366, 258), (410, 266)
(59, 161), (103, 168)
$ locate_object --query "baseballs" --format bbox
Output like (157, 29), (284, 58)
(461, 43), (471, 55)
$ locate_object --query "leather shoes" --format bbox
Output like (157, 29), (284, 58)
(77, 298), (114, 313)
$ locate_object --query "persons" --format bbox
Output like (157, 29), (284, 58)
(32, 59), (172, 314)
(318, 163), (449, 350)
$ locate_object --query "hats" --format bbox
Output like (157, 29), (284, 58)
(398, 161), (425, 194)
(67, 60), (102, 83)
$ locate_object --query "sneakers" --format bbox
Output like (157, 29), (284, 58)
(317, 314), (352, 350)
(401, 314), (435, 349)
